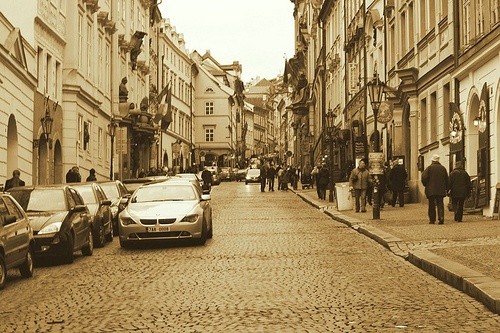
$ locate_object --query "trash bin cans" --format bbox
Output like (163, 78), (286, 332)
(335, 182), (353, 211)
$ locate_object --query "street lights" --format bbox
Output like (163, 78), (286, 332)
(365, 71), (387, 219)
(324, 108), (337, 204)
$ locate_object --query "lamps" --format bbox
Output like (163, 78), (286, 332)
(33, 112), (53, 149)
(449, 123), (460, 139)
(473, 115), (481, 127)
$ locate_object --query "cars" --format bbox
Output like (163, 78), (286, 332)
(117, 178), (213, 248)
(3, 184), (94, 264)
(93, 180), (132, 235)
(245, 169), (262, 184)
(60, 182), (115, 247)
(122, 165), (248, 196)
(0, 191), (35, 290)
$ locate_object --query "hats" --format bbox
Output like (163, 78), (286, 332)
(431, 154), (440, 161)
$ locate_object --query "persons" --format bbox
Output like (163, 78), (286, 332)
(348, 159), (408, 213)
(124, 162), (212, 191)
(421, 154), (448, 225)
(447, 161), (471, 223)
(3, 170), (25, 193)
(260, 162), (328, 201)
(119, 77), (129, 103)
(65, 166), (82, 183)
(86, 168), (96, 181)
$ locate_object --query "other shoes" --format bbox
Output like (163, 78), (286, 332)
(429, 221), (434, 224)
(361, 210), (367, 212)
(454, 218), (463, 222)
(355, 210), (359, 212)
(438, 222), (444, 224)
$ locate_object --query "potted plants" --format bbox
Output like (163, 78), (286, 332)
(398, 186), (411, 204)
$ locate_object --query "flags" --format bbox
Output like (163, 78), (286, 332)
(283, 59), (291, 84)
(157, 86), (172, 131)
